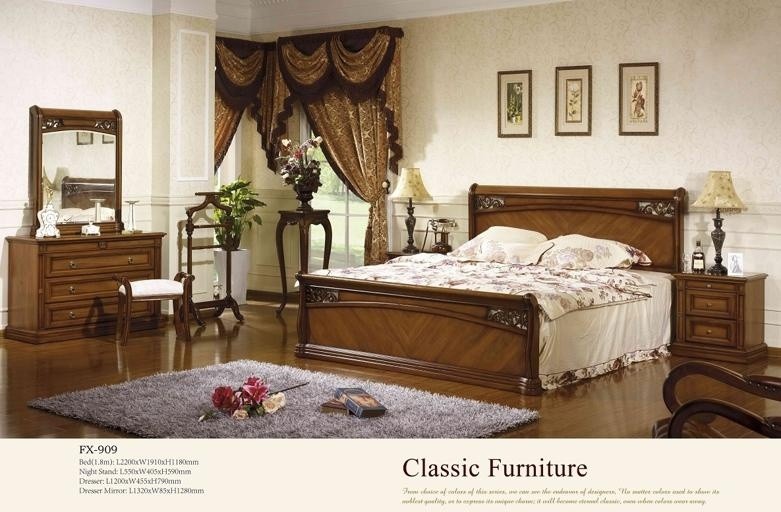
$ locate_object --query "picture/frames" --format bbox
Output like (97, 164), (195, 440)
(619, 62), (659, 136)
(728, 252), (744, 277)
(498, 70), (532, 138)
(556, 65), (592, 136)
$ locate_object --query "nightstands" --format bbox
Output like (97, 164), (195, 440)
(669, 269), (768, 363)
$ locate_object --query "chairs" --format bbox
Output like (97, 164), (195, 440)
(653, 360), (781, 439)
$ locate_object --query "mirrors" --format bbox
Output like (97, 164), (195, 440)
(28, 105), (125, 236)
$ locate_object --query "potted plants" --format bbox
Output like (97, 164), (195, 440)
(213, 177), (267, 307)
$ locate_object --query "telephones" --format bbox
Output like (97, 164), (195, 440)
(421, 218), (458, 254)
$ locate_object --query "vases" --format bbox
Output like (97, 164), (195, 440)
(293, 181), (322, 211)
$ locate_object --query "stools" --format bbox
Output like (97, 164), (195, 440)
(275, 209), (332, 313)
(113, 271), (195, 346)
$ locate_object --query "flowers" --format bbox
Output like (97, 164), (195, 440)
(197, 374), (309, 424)
(273, 134), (323, 186)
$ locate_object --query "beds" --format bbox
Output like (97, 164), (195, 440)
(293, 183), (688, 397)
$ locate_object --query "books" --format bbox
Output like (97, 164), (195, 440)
(319, 398), (352, 416)
(331, 387), (388, 418)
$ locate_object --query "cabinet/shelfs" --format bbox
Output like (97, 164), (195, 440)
(4, 231), (167, 344)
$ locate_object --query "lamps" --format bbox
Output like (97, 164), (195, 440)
(688, 170), (747, 275)
(388, 168), (433, 255)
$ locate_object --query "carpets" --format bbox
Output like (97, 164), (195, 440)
(27, 360), (540, 440)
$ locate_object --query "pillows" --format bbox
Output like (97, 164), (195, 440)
(446, 226), (549, 265)
(532, 233), (652, 270)
